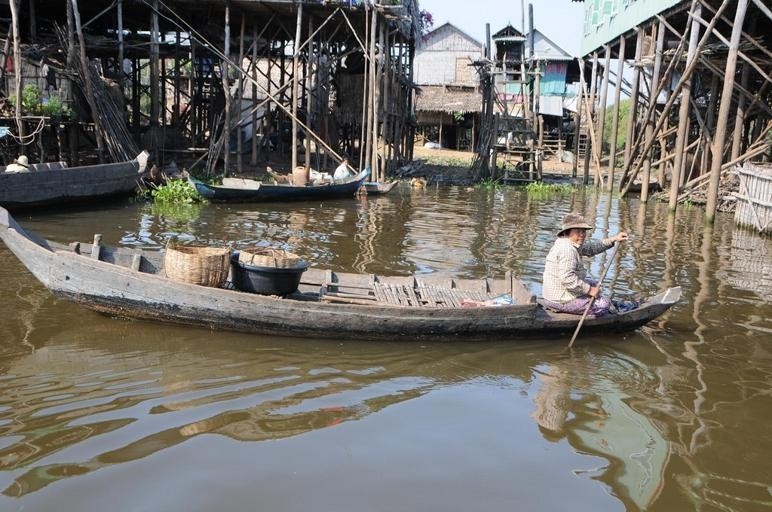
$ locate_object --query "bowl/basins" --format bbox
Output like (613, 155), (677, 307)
(228, 250), (311, 296)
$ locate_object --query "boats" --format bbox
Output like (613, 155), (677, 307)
(183, 167), (372, 204)
(1, 149), (151, 207)
(0, 207), (682, 343)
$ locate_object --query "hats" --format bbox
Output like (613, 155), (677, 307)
(556, 213), (594, 236)
(13, 154), (31, 168)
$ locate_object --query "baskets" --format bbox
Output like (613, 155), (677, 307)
(164, 238), (231, 289)
(238, 248), (301, 268)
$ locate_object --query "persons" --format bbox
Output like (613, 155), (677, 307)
(541, 213), (630, 318)
(333, 155), (355, 179)
(4, 154), (29, 174)
